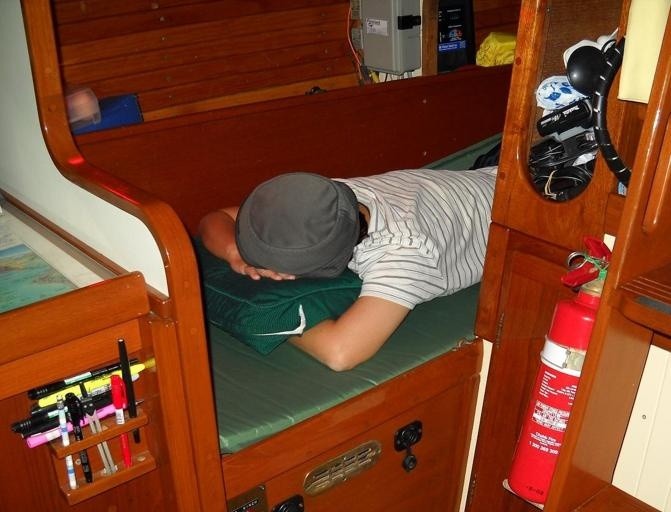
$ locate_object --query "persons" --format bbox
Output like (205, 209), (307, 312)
(198, 165), (498, 374)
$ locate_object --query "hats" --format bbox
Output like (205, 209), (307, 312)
(235, 171), (361, 279)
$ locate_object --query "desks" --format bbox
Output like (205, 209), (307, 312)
(1, 189), (227, 511)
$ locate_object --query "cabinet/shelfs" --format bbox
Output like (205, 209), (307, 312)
(476, 0), (670, 511)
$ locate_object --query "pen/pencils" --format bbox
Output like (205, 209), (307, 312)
(117, 338), (141, 444)
(65, 392), (93, 483)
(56, 394), (77, 490)
(10, 357), (146, 448)
(110, 374), (131, 469)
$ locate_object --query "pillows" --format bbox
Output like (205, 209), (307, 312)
(189, 234), (363, 356)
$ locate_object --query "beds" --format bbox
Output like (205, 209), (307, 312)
(66, 131), (522, 512)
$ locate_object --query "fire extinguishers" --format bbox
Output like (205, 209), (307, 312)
(507, 233), (617, 506)
